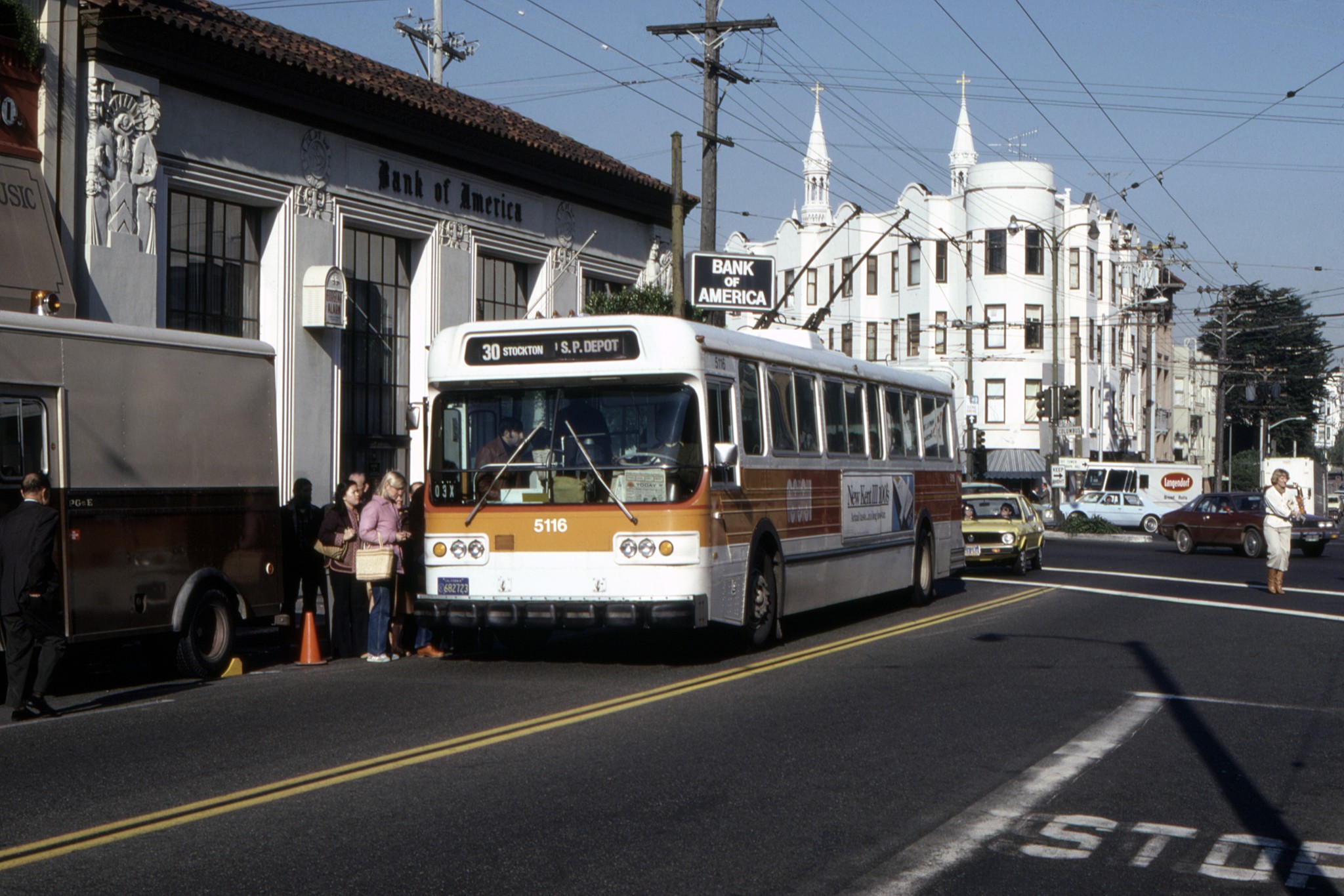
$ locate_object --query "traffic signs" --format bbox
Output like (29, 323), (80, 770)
(1051, 466), (1066, 488)
(1058, 458), (1089, 472)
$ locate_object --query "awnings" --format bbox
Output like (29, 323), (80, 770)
(983, 449), (1046, 479)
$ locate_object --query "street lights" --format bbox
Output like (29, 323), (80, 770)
(1099, 297), (1170, 480)
(1267, 416), (1309, 457)
(1007, 214), (1100, 530)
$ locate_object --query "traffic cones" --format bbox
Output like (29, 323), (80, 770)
(292, 611), (327, 667)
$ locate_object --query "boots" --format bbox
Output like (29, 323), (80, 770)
(1268, 567), (1277, 593)
(1275, 570), (1285, 594)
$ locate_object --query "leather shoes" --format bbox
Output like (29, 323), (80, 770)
(418, 644), (444, 658)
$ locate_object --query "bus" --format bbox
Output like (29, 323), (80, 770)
(404, 206), (963, 653)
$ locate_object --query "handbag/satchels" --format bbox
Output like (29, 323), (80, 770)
(313, 508), (349, 561)
(356, 532), (394, 580)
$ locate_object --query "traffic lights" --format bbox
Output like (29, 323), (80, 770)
(1059, 388), (1081, 417)
(977, 430), (985, 451)
(1036, 390), (1052, 417)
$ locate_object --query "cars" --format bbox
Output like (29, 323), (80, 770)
(960, 481), (1054, 576)
(1328, 484), (1344, 523)
(1159, 491), (1340, 559)
(1042, 491), (1163, 534)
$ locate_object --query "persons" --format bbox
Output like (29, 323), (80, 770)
(279, 478), (321, 640)
(962, 504), (976, 521)
(318, 481), (369, 661)
(1263, 469), (1301, 594)
(0, 473), (66, 721)
(348, 471), (373, 513)
(1000, 503), (1016, 520)
(1106, 497), (1112, 505)
(553, 389), (613, 497)
(1032, 483), (1050, 503)
(474, 416), (524, 501)
(389, 482), (444, 658)
(1219, 500), (1233, 513)
(358, 471), (413, 663)
(650, 416), (702, 484)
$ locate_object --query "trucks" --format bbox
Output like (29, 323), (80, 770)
(1260, 457), (1329, 518)
(1077, 462), (1203, 512)
(0, 290), (276, 676)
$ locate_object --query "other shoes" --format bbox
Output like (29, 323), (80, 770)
(391, 646), (412, 657)
(27, 695), (61, 716)
(386, 652), (399, 661)
(367, 652), (391, 662)
(12, 708), (40, 720)
